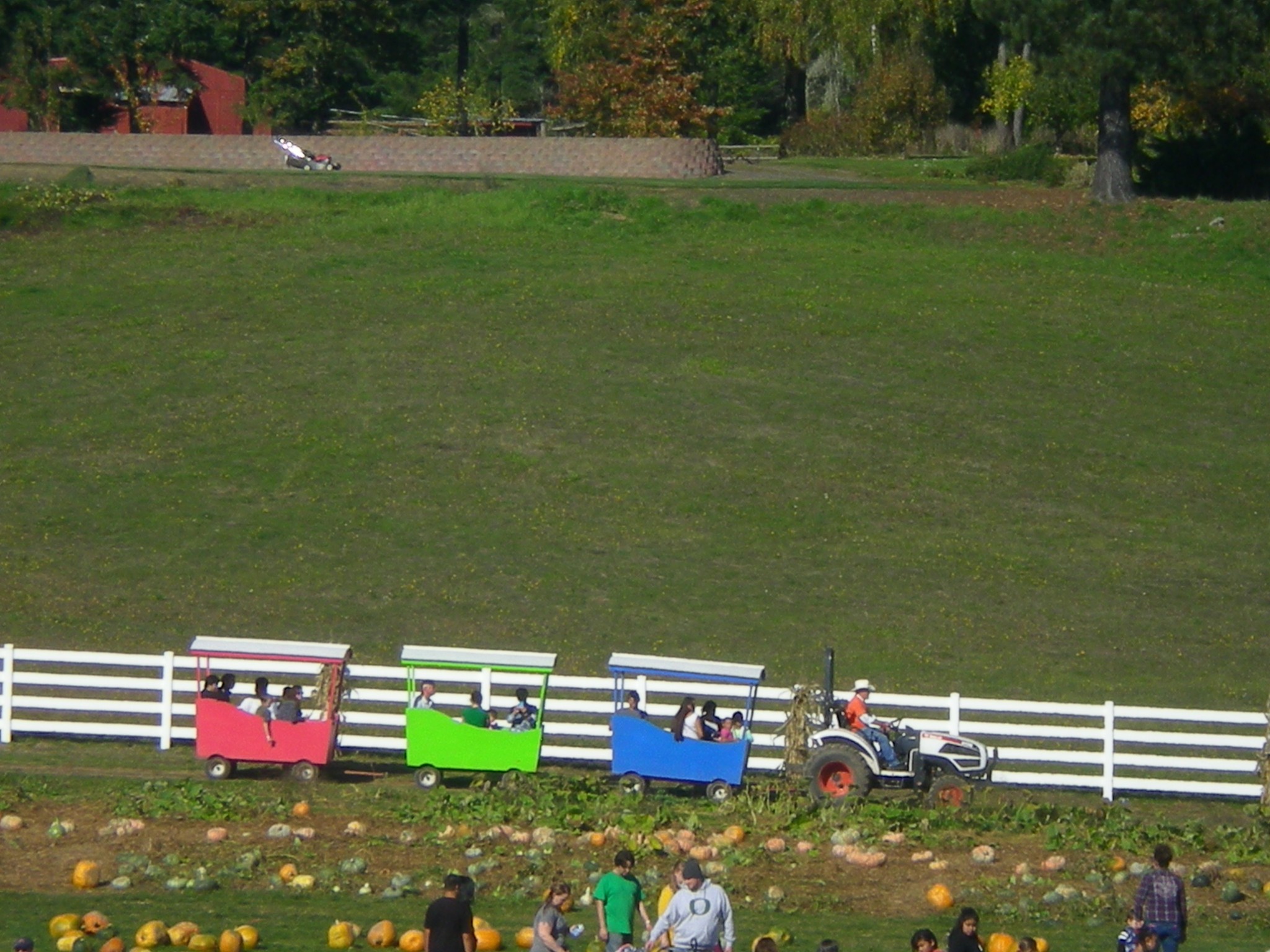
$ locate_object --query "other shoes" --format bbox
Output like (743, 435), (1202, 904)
(890, 762), (904, 769)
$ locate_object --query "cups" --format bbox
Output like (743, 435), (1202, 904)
(570, 924), (584, 937)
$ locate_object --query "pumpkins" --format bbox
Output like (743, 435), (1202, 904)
(0, 802), (556, 952)
(574, 824), (933, 951)
(925, 845), (1270, 952)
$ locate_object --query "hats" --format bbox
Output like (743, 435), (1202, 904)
(683, 861), (702, 879)
(850, 679), (876, 691)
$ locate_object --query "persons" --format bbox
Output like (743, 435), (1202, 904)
(609, 690), (754, 743)
(422, 850), (1040, 952)
(844, 679), (906, 769)
(201, 672), (304, 743)
(413, 680), (537, 731)
(1115, 843), (1188, 952)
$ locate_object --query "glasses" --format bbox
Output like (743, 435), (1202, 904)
(297, 691), (304, 695)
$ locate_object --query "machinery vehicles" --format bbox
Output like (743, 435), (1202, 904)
(784, 649), (1000, 810)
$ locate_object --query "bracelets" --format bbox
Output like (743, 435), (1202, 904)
(646, 920), (651, 924)
